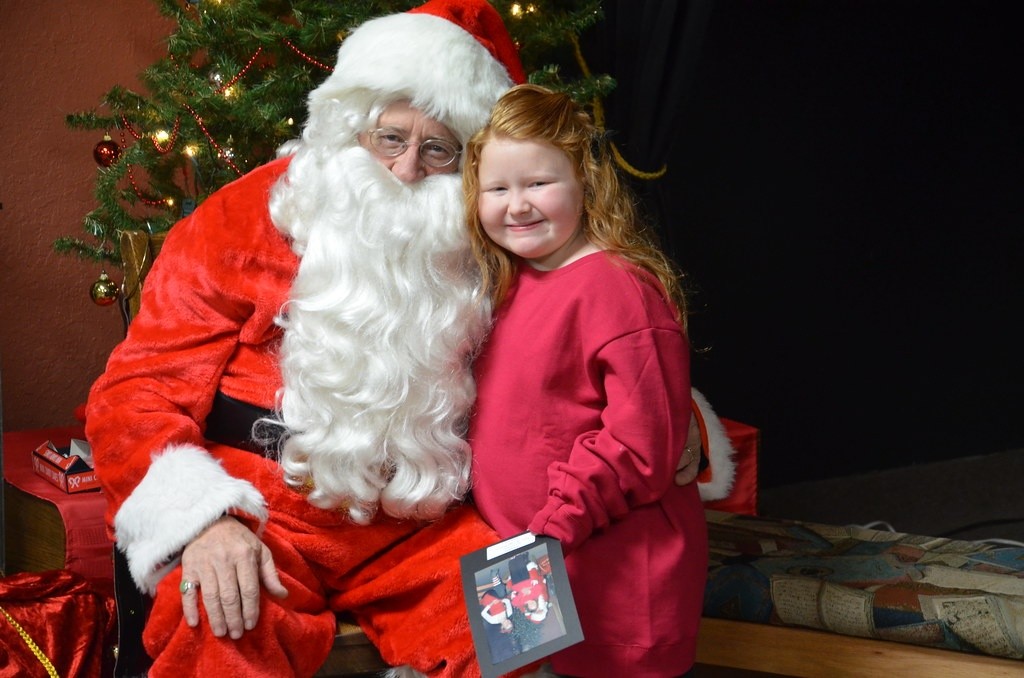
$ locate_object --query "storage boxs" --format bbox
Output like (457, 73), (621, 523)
(30, 438), (101, 494)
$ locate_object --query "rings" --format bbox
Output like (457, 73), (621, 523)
(180, 579), (198, 593)
(687, 447), (696, 462)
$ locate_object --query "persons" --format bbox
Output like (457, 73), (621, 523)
(84, 1), (763, 678)
(461, 85), (708, 678)
(479, 553), (549, 634)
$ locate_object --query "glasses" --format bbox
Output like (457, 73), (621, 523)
(363, 126), (466, 167)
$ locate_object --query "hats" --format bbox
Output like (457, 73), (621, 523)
(307, 0), (528, 148)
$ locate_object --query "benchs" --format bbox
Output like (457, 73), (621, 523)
(694, 509), (1024, 678)
